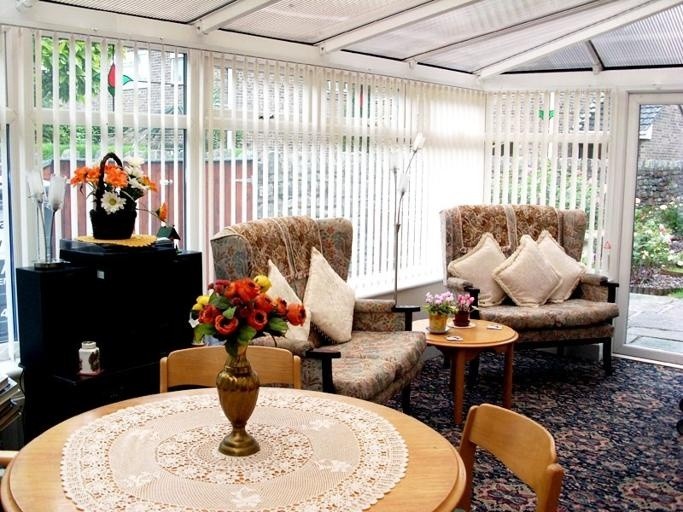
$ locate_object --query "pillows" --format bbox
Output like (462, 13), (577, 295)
(262, 245), (359, 344)
(447, 231), (589, 309)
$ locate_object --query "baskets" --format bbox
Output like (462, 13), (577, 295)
(89, 152), (138, 239)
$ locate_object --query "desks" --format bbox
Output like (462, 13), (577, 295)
(1, 385), (467, 512)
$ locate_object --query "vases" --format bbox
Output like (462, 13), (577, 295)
(428, 310), (471, 333)
(213, 339), (261, 457)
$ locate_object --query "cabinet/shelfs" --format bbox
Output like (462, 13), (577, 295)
(10, 248), (203, 444)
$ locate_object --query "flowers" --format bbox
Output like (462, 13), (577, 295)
(68, 155), (169, 228)
(186, 276), (307, 348)
(422, 287), (474, 316)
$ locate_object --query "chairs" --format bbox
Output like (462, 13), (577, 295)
(208, 213), (428, 414)
(438, 201), (620, 382)
(453, 403), (565, 512)
(157, 342), (301, 393)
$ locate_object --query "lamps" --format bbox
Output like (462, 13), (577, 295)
(388, 132), (428, 307)
(23, 166), (67, 270)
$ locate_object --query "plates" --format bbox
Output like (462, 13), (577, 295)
(424, 326), (450, 334)
(446, 323), (475, 329)
